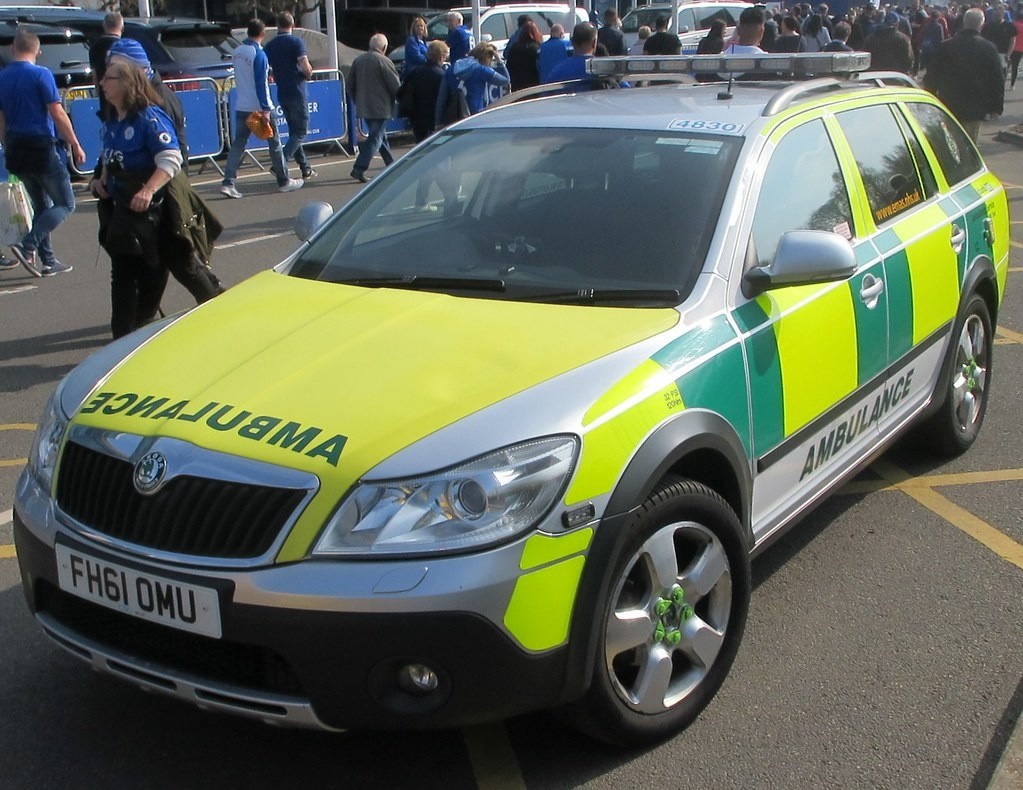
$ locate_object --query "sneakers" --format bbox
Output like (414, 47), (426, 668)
(11, 242), (41, 277)
(0, 254), (19, 270)
(278, 178), (304, 192)
(43, 257), (73, 277)
(220, 185), (242, 198)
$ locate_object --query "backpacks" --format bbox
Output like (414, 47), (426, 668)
(440, 80), (471, 129)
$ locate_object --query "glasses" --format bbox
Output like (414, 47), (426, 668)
(103, 75), (121, 82)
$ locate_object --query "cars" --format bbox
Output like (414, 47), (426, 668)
(0, 6), (368, 144)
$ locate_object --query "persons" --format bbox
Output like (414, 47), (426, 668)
(262, 10), (319, 180)
(0, 30), (86, 277)
(395, 40), (466, 213)
(219, 19), (305, 198)
(404, 0), (1023, 146)
(86, 39), (226, 339)
(346, 34), (402, 184)
(87, 12), (125, 193)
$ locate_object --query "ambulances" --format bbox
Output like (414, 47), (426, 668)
(12, 51), (1009, 746)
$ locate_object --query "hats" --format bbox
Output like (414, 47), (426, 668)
(105, 37), (151, 69)
(885, 10), (899, 26)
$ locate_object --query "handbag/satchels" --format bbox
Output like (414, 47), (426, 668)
(1, 174), (35, 245)
(104, 193), (161, 262)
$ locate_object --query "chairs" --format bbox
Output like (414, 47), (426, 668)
(550, 139), (724, 231)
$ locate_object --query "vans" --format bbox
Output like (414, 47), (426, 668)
(619, 3), (760, 56)
(389, 5), (590, 63)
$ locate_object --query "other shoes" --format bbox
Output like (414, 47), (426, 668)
(269, 166), (276, 177)
(350, 168), (370, 183)
(415, 203), (437, 213)
(302, 169), (318, 179)
